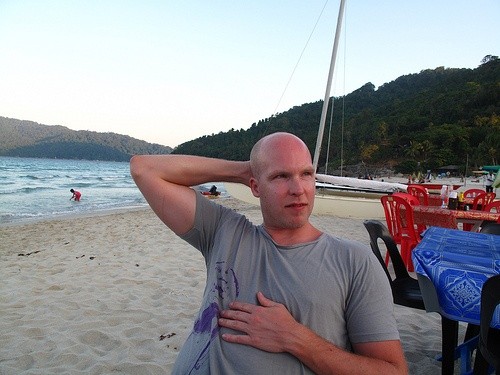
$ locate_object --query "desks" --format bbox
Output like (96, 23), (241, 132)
(399, 204), (500, 234)
(419, 194), (500, 211)
(411, 226), (500, 375)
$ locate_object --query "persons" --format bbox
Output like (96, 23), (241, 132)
(130, 132), (410, 375)
(485, 169), (495, 192)
(408, 170), (421, 183)
(209, 185), (217, 195)
(70, 189), (81, 201)
(426, 170), (434, 183)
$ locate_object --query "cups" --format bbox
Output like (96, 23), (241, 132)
(459, 201), (466, 210)
(465, 205), (469, 210)
(449, 198), (458, 210)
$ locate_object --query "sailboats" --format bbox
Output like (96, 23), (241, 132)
(301, 1), (409, 199)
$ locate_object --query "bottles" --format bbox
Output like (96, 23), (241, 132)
(441, 185), (463, 208)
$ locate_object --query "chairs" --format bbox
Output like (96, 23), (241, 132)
(363, 186), (500, 375)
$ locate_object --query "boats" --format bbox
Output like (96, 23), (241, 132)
(199, 191), (221, 199)
